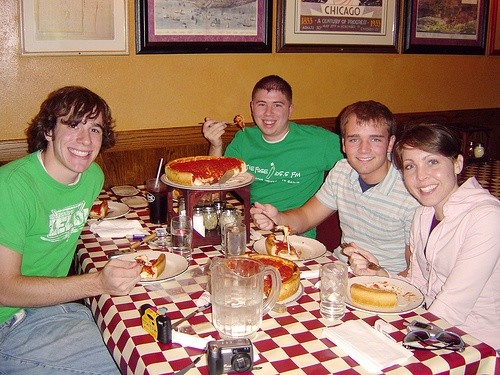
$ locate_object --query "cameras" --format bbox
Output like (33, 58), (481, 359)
(207, 338), (254, 375)
(138, 304), (172, 344)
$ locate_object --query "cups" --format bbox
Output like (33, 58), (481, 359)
(170, 216), (191, 259)
(224, 222), (246, 258)
(319, 262), (348, 319)
(147, 178), (168, 224)
(209, 256), (282, 342)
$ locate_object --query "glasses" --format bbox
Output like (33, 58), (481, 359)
(403, 330), (465, 350)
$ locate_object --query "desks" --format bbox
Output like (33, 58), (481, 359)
(457, 157), (500, 201)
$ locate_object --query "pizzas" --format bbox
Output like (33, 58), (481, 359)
(205, 254), (301, 302)
(165, 155), (247, 185)
(134, 253), (166, 279)
(89, 199), (109, 219)
(233, 115), (245, 130)
(265, 225), (299, 258)
(350, 282), (397, 307)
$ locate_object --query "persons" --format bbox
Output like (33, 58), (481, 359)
(342, 122), (500, 352)
(249, 99), (423, 279)
(0, 85), (146, 375)
(201, 74), (345, 240)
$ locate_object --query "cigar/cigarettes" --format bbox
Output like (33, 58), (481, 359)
(130, 231), (157, 249)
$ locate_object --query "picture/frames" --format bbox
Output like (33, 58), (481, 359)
(277, 0), (400, 53)
(488, 0), (500, 56)
(401, 0), (489, 55)
(18, 0), (129, 55)
(135, 0), (271, 52)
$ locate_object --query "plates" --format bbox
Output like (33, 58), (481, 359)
(276, 282), (305, 307)
(111, 250), (190, 285)
(161, 172), (255, 191)
(252, 235), (327, 262)
(345, 276), (426, 316)
(94, 201), (130, 220)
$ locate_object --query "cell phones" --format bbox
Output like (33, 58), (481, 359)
(402, 319), (443, 337)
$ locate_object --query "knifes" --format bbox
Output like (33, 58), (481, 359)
(172, 304), (211, 330)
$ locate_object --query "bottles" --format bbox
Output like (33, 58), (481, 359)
(220, 208), (241, 253)
(214, 201), (225, 227)
(177, 196), (186, 217)
(203, 204), (217, 231)
(191, 206), (205, 237)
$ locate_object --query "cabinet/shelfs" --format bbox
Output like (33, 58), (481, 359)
(167, 185), (250, 249)
